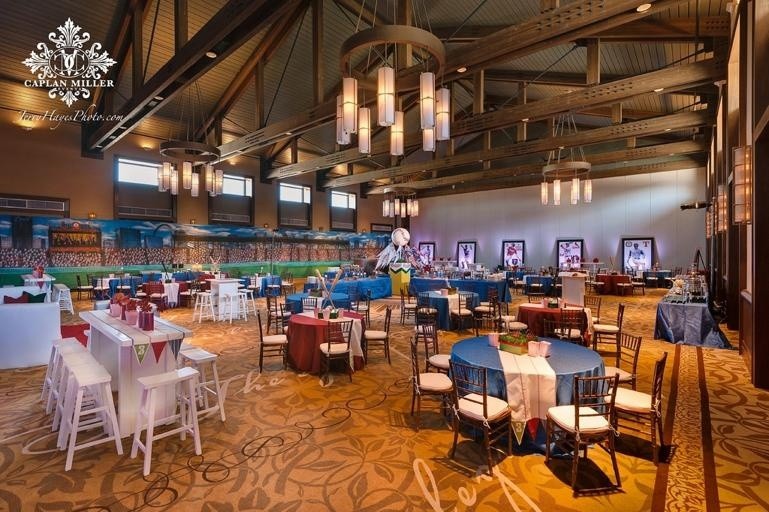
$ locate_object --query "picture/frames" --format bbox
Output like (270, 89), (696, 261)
(621, 237), (654, 274)
(502, 240), (525, 271)
(457, 240), (476, 268)
(418, 241), (435, 260)
(557, 239), (584, 272)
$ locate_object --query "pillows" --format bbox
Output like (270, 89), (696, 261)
(4, 291), (47, 303)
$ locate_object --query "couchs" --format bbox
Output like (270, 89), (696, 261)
(0, 302), (61, 370)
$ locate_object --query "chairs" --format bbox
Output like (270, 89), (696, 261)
(76, 268), (256, 324)
(256, 271), (393, 385)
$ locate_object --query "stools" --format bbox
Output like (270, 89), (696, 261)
(52, 283), (74, 316)
(40, 329), (226, 477)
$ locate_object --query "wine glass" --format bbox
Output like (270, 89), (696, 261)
(37, 280), (45, 293)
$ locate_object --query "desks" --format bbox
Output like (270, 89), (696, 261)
(20, 273), (56, 302)
(654, 274), (735, 349)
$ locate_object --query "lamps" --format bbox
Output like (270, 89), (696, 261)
(541, 89), (593, 205)
(336, 1), (452, 157)
(156, 79), (224, 198)
(704, 145), (729, 240)
(383, 152), (419, 219)
(732, 144), (752, 225)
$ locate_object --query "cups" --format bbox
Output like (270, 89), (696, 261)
(314, 306), (344, 320)
(488, 333), (498, 346)
(528, 340), (552, 358)
(487, 273), (503, 280)
(144, 311), (154, 331)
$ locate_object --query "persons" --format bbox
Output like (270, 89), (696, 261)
(505, 243), (521, 267)
(560, 241), (580, 270)
(627, 243), (646, 271)
(424, 246), (431, 262)
(460, 244), (472, 268)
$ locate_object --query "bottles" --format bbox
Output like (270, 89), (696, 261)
(543, 297), (567, 308)
(418, 265), (446, 279)
